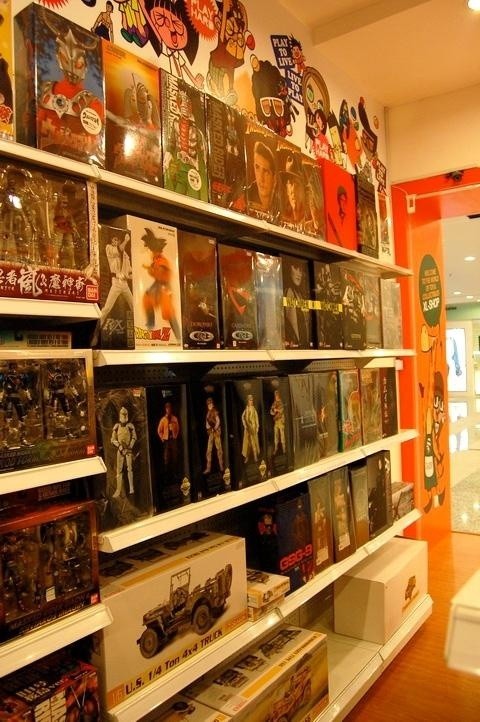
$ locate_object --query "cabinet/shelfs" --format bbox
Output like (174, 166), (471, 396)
(1, 140), (435, 722)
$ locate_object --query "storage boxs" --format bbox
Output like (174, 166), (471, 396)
(87, 528), (250, 711)
(181, 623), (332, 721)
(334, 536), (428, 646)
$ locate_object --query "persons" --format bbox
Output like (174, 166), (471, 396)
(0, 521), (101, 643)
(91, 232), (134, 345)
(249, 142), (326, 239)
(0, 170), (81, 268)
(337, 186), (348, 219)
(0, 360), (80, 449)
(285, 260), (308, 345)
